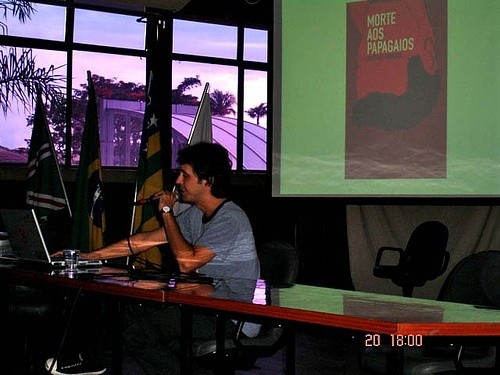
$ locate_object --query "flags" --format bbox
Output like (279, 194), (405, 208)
(128, 74), (171, 272)
(173, 90), (212, 202)
(71, 76), (105, 254)
(26, 87), (73, 237)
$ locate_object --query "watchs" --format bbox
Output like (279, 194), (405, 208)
(160, 205), (174, 215)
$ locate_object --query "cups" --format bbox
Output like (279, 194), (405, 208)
(63, 250), (80, 272)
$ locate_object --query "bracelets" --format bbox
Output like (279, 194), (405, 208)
(126, 237), (136, 256)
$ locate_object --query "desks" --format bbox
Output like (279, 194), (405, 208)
(0, 231), (500, 375)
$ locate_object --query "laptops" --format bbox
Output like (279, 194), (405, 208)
(0, 209), (107, 267)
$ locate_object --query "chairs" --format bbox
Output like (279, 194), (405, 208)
(374, 220), (450, 296)
(182, 233), (298, 375)
(395, 252), (500, 375)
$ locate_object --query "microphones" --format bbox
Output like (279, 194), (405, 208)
(135, 192), (182, 205)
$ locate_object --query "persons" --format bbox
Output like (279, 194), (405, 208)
(45, 141), (260, 375)
